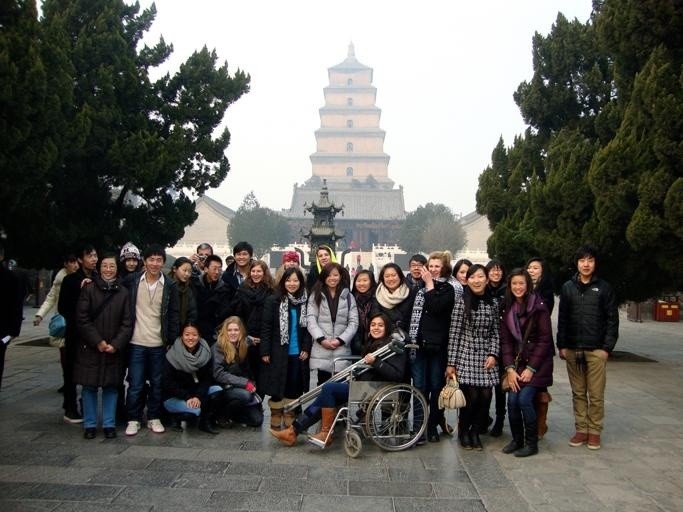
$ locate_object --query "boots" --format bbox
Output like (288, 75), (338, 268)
(536, 392), (552, 441)
(310, 408), (337, 447)
(284, 398), (296, 428)
(268, 400), (284, 430)
(269, 425), (297, 446)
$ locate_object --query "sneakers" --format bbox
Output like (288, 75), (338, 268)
(587, 433), (601, 450)
(569, 432), (587, 447)
(146, 418), (165, 434)
(428, 425), (439, 442)
(216, 416), (235, 430)
(417, 436), (426, 446)
(125, 420), (141, 435)
(442, 421), (453, 434)
(63, 407), (82, 422)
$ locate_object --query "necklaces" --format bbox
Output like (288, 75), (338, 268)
(142, 271), (163, 308)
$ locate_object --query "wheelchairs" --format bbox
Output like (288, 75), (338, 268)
(307, 345), (428, 457)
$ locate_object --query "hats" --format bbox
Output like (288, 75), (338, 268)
(282, 251), (300, 263)
(120, 241), (140, 263)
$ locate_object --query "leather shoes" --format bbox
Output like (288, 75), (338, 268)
(513, 444), (539, 456)
(491, 425), (504, 434)
(470, 430), (483, 451)
(105, 427), (116, 439)
(85, 427), (97, 439)
(200, 409), (220, 434)
(459, 431), (474, 451)
(502, 440), (522, 453)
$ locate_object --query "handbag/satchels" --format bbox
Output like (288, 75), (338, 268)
(438, 374), (465, 410)
(49, 313), (67, 338)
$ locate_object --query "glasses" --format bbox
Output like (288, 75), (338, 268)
(409, 263), (425, 269)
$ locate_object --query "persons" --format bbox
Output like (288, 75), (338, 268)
(113, 242), (144, 282)
(344, 263), (350, 274)
(0, 238), (24, 390)
(352, 270), (376, 356)
(58, 246), (97, 425)
(231, 261), (277, 401)
(32, 254), (80, 395)
(373, 262), (412, 334)
(263, 312), (410, 447)
(210, 316), (265, 429)
(221, 241), (272, 288)
(307, 261), (360, 389)
(368, 262), (375, 274)
(120, 244), (182, 435)
(160, 324), (225, 433)
(403, 254), (428, 314)
(258, 266), (312, 432)
(188, 242), (213, 276)
(190, 254), (233, 344)
(556, 246), (620, 451)
(451, 258), (472, 299)
(224, 256), (233, 266)
(350, 267), (356, 279)
(168, 257), (197, 330)
(497, 268), (554, 458)
(308, 245), (339, 293)
(484, 258), (510, 310)
(355, 262), (363, 274)
(273, 249), (309, 288)
(443, 263), (500, 452)
(75, 253), (132, 439)
(405, 250), (455, 445)
(524, 258), (558, 441)
